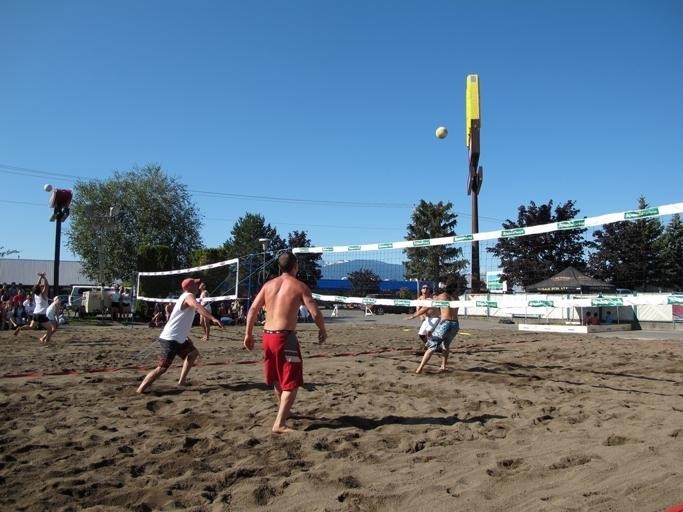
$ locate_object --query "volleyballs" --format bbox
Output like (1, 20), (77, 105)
(436, 127), (448, 139)
(44, 184), (52, 192)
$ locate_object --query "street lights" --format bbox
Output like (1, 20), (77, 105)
(258, 236), (271, 284)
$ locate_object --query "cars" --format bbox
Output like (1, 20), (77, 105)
(361, 294), (418, 314)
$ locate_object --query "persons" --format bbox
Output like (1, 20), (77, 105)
(110, 283), (314, 327)
(243, 253), (329, 435)
(0, 282), (68, 344)
(413, 282), (445, 356)
(401, 276), (460, 375)
(197, 282), (212, 342)
(13, 270), (54, 344)
(134, 278), (228, 394)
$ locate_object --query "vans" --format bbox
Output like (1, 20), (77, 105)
(67, 284), (134, 317)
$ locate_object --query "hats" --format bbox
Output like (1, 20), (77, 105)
(181, 278), (200, 291)
